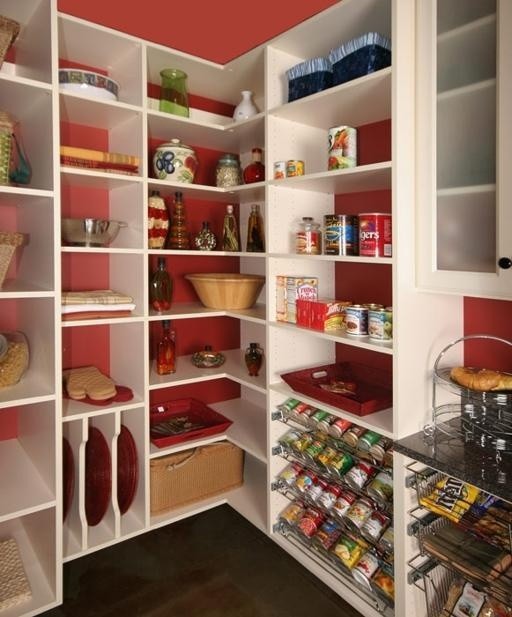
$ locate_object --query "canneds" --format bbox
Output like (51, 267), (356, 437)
(273, 160), (305, 180)
(323, 212), (392, 258)
(345, 303), (393, 343)
(278, 399), (395, 610)
(327, 125), (358, 171)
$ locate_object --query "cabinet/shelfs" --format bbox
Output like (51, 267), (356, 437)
(0, 1), (57, 617)
(57, 6), (144, 579)
(415, 1), (511, 303)
(393, 416), (511, 616)
(264, 1), (464, 616)
(145, 29), (334, 538)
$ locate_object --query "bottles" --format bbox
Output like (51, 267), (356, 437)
(153, 139), (198, 183)
(245, 343), (264, 376)
(0, 110), (14, 185)
(149, 257), (174, 312)
(157, 320), (176, 375)
(216, 148), (265, 188)
(232, 91), (259, 122)
(191, 345), (226, 368)
(296, 214), (322, 255)
(148, 191), (265, 252)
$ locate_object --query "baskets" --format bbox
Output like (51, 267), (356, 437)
(0, 15), (21, 73)
(0, 231), (25, 290)
(0, 108), (17, 186)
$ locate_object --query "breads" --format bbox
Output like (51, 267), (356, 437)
(450, 367), (512, 392)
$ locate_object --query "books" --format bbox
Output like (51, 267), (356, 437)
(59, 144), (139, 176)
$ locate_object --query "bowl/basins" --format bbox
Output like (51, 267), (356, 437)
(59, 68), (120, 102)
(61, 218), (128, 247)
(184, 273), (266, 309)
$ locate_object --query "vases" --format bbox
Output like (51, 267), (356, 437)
(148, 189), (168, 248)
(242, 145), (265, 184)
(169, 191), (190, 248)
(231, 89), (260, 124)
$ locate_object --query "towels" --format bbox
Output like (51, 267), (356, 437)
(60, 288), (135, 320)
